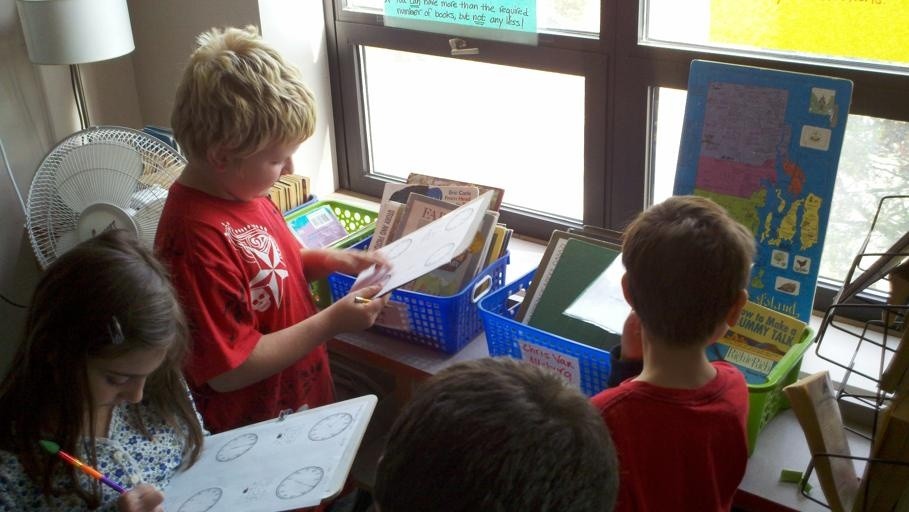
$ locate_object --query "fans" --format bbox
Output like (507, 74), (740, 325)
(26, 125), (188, 272)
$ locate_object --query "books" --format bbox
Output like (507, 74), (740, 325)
(366, 172), (513, 337)
(268, 172), (310, 214)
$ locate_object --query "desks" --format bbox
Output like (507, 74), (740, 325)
(317, 312), (894, 512)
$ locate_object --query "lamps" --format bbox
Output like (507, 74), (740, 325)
(15, 2), (135, 131)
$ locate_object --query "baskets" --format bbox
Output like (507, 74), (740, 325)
(281, 195), (511, 355)
(477, 267), (816, 457)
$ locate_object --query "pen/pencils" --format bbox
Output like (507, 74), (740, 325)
(355, 296), (371, 304)
(39, 440), (127, 494)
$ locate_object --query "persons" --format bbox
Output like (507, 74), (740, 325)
(0, 230), (212, 511)
(373, 355), (622, 511)
(589, 195), (749, 512)
(153, 25), (392, 433)
